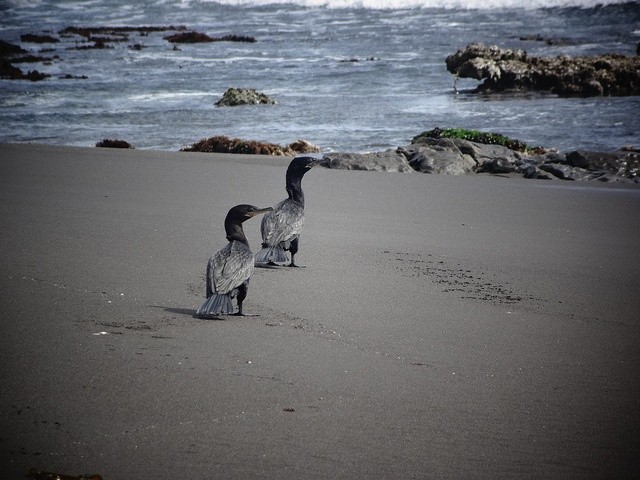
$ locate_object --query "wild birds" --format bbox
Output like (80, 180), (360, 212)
(196, 204), (274, 316)
(254, 156), (332, 268)
